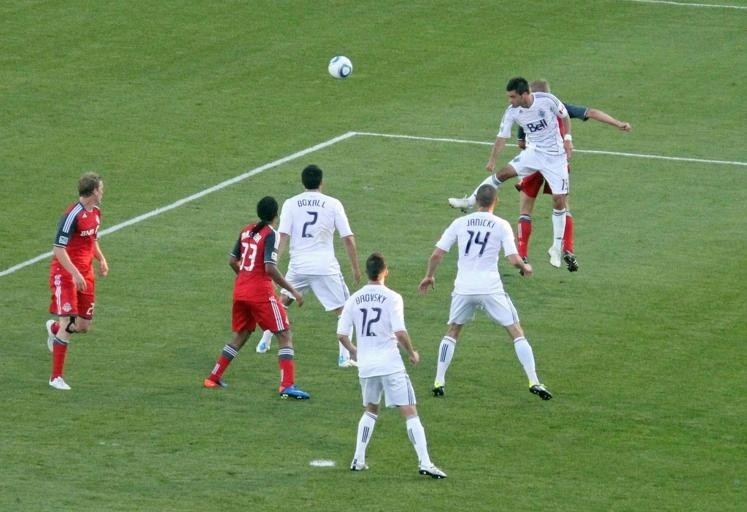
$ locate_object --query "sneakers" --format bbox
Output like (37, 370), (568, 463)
(256, 342), (271, 353)
(548, 246), (561, 267)
(563, 252), (579, 272)
(529, 384), (553, 401)
(432, 382), (445, 397)
(518, 255), (527, 275)
(204, 379), (228, 388)
(48, 375), (71, 390)
(279, 383), (310, 399)
(350, 459), (369, 471)
(418, 462), (447, 479)
(448, 194), (473, 213)
(338, 359), (358, 367)
(45, 319), (56, 353)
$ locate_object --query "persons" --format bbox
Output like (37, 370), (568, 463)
(46, 171), (109, 390)
(447, 77), (573, 267)
(204, 196), (313, 400)
(419, 185), (553, 401)
(336, 251), (447, 479)
(516, 79), (632, 275)
(257, 164), (360, 367)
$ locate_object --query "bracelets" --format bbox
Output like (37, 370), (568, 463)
(563, 134), (572, 141)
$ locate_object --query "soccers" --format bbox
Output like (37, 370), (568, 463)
(327, 57), (351, 78)
